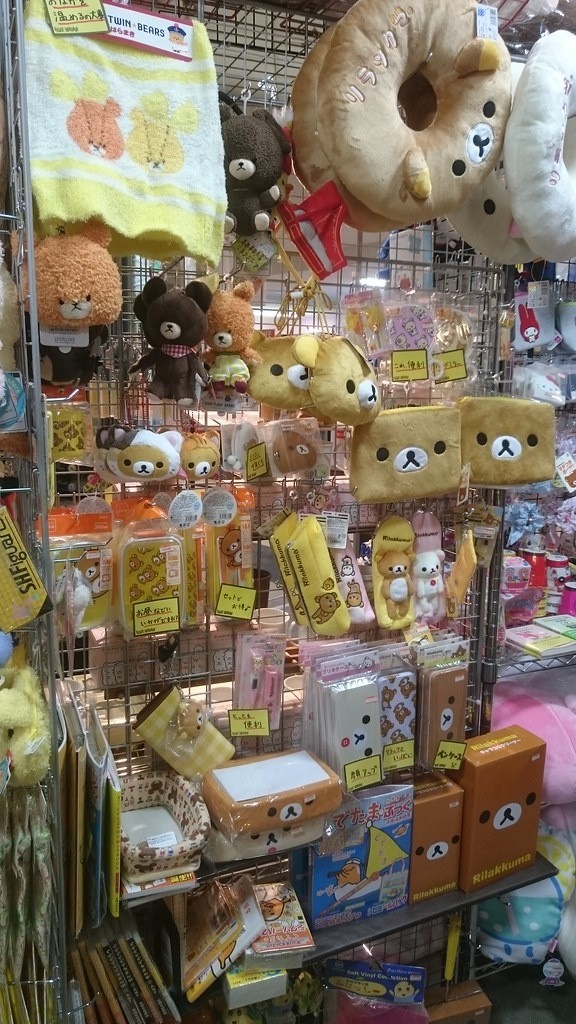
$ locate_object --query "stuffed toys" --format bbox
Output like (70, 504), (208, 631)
(206, 278), (261, 393)
(95, 424), (221, 484)
(219, 101), (295, 236)
(1, 667), (50, 788)
(132, 278), (211, 406)
(11, 220), (123, 388)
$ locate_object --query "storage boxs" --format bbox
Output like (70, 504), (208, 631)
(445, 723), (547, 894)
(223, 964), (290, 1009)
(404, 769), (465, 905)
(424, 981), (492, 1024)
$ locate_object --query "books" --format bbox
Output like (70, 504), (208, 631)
(73, 869), (315, 1024)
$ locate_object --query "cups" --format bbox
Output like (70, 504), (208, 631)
(519, 546), (576, 618)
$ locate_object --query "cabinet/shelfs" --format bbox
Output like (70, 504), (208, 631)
(0, 0), (576, 1024)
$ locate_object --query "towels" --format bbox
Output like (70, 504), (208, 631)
(23, 0), (225, 266)
